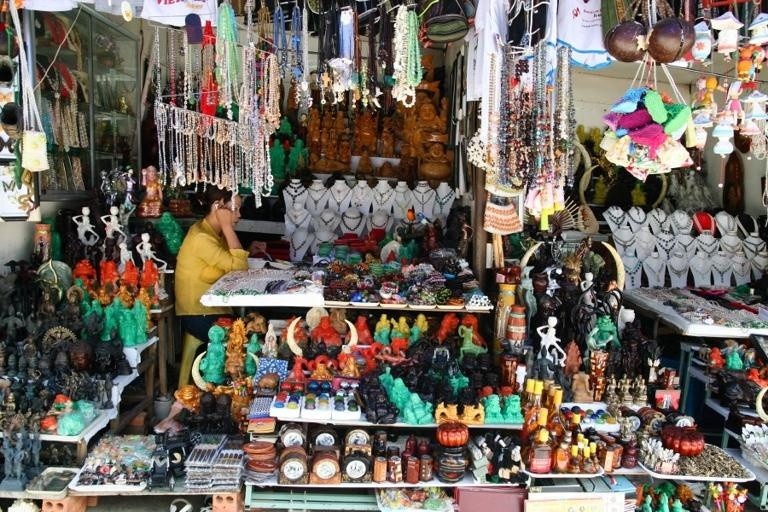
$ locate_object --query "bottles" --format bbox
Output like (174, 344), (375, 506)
(376, 432), (430, 456)
(520, 377), (599, 474)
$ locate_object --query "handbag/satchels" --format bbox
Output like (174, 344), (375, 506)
(21, 128), (50, 174)
(599, 85), (696, 183)
(483, 201), (524, 237)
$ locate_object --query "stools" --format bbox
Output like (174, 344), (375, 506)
(178, 332), (206, 394)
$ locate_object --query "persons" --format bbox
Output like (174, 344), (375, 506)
(172, 184), (251, 349)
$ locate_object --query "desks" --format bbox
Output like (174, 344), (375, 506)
(622, 286), (768, 423)
(11, 306), (171, 467)
(200, 256), (498, 324)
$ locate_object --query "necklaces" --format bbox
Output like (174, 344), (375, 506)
(33, 9), (90, 125)
(282, 178), (458, 257)
(145, 0), (422, 208)
(606, 207), (768, 295)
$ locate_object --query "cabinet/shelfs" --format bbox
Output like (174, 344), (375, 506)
(0, 1), (146, 226)
